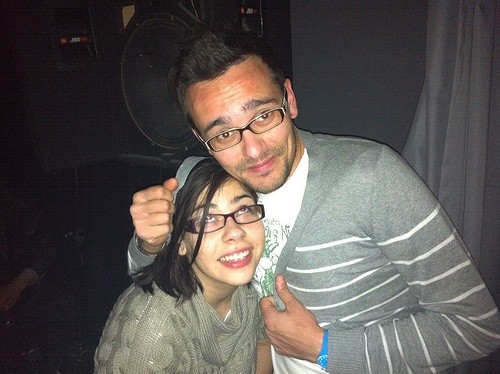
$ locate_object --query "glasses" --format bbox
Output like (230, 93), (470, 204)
(183, 205), (265, 233)
(190, 87), (287, 153)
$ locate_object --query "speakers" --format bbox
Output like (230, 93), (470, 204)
(67, 0), (206, 159)
(59, 162), (182, 374)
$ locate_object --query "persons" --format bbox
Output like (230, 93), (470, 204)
(128, 31), (500, 374)
(95, 157), (274, 374)
(0, 209), (57, 311)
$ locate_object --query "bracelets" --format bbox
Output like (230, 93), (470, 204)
(317, 329), (328, 369)
